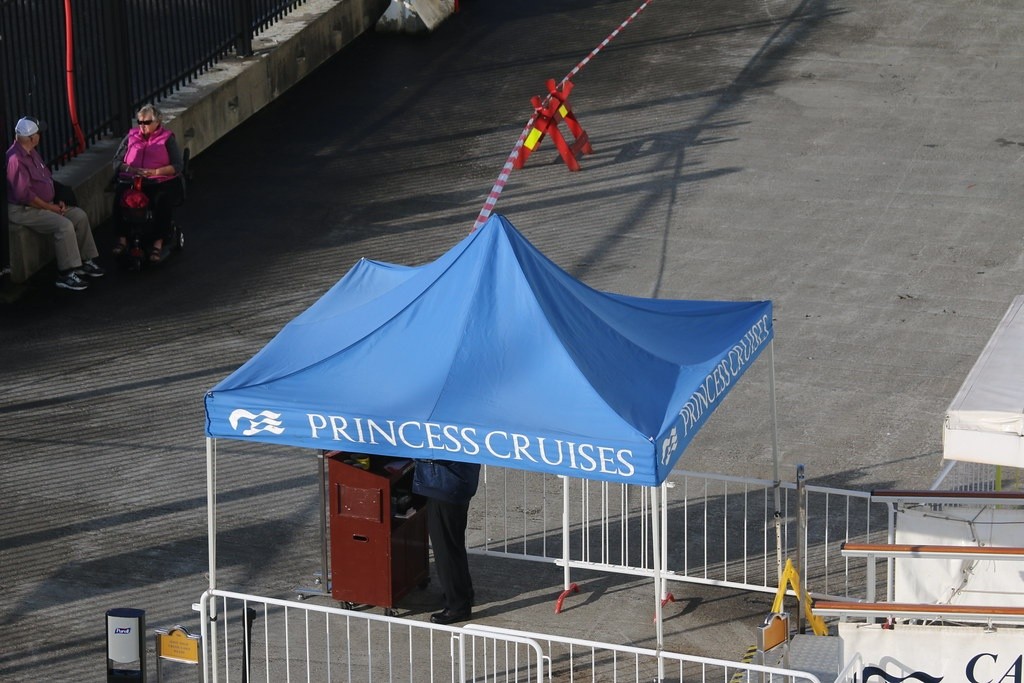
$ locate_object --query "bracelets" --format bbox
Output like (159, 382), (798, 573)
(125, 166), (129, 172)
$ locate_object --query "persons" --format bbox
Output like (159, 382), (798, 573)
(6, 115), (104, 291)
(411, 459), (482, 626)
(112, 104), (183, 262)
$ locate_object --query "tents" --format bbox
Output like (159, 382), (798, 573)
(202, 215), (782, 683)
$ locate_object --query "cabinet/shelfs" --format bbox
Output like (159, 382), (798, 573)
(325, 450), (429, 616)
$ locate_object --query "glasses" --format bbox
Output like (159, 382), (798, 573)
(137, 119), (156, 125)
(36, 131), (43, 136)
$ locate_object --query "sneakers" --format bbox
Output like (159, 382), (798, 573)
(55, 271), (89, 290)
(73, 260), (105, 277)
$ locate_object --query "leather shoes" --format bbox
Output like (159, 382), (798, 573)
(430, 606), (472, 624)
(443, 591), (475, 601)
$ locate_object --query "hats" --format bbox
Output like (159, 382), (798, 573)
(14, 116), (48, 136)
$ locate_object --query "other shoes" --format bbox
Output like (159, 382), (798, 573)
(149, 246), (161, 261)
(112, 243), (129, 255)
